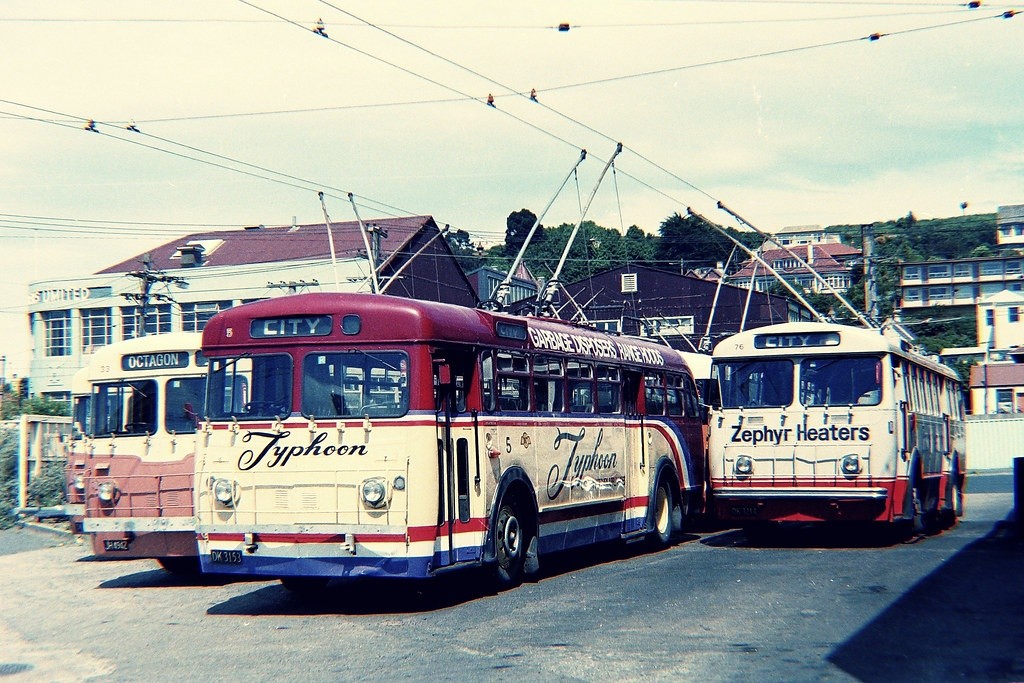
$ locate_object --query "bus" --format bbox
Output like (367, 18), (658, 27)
(187, 143), (708, 588)
(63, 191), (521, 579)
(685, 200), (968, 537)
